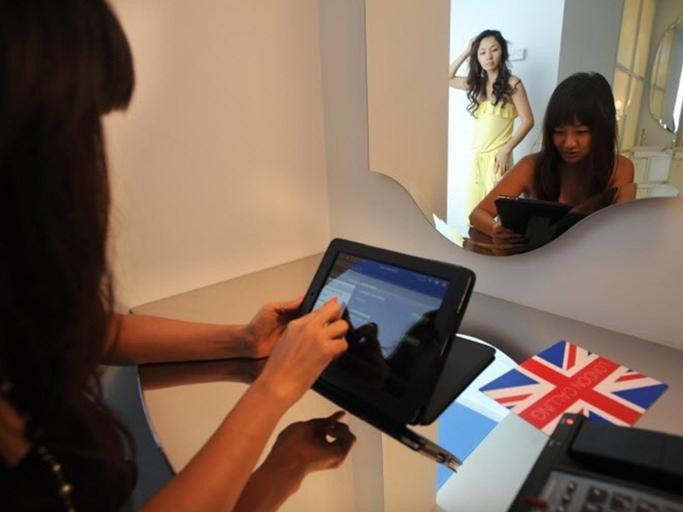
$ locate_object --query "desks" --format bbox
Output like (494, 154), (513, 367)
(128, 254), (683, 512)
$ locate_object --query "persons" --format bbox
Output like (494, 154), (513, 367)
(0, 2), (348, 512)
(444, 29), (535, 223)
(469, 71), (635, 243)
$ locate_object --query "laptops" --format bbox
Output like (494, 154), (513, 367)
(495, 195), (583, 244)
(297, 236), (496, 426)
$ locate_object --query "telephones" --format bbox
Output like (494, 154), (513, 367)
(507, 410), (683, 512)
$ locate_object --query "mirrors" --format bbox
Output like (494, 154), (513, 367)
(647, 15), (682, 135)
(364, 2), (683, 257)
